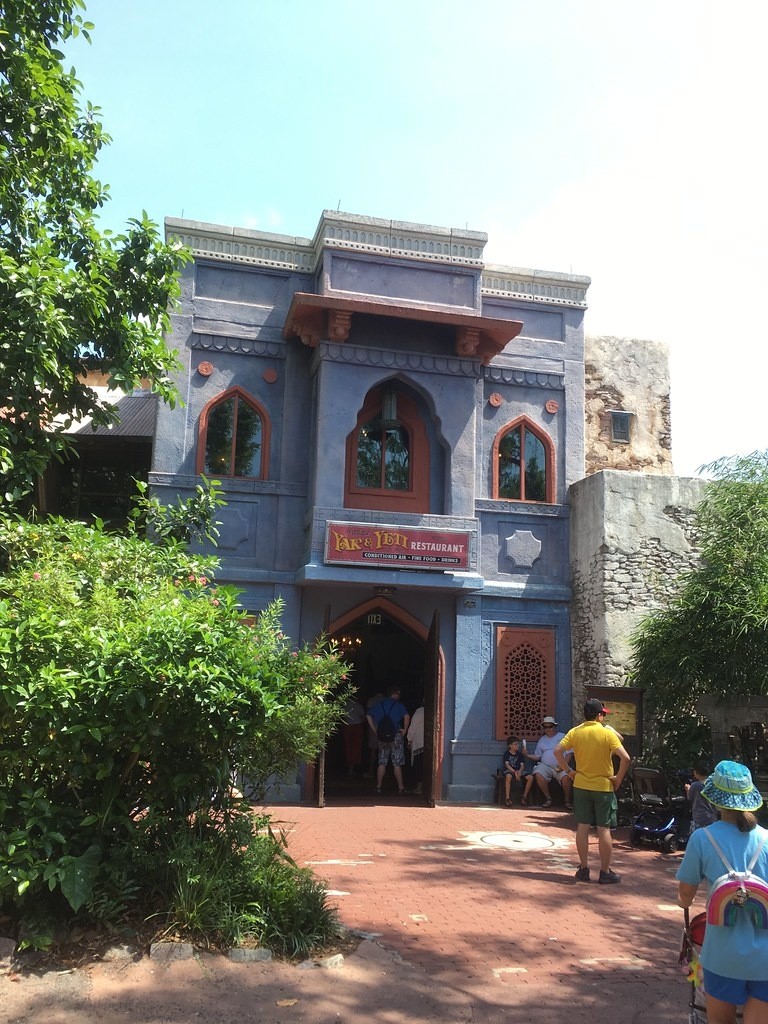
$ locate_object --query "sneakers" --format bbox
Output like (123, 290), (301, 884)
(599, 868), (621, 884)
(575, 865), (591, 881)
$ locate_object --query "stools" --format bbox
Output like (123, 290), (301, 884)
(496, 776), (532, 806)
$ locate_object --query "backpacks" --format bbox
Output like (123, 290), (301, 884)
(702, 827), (768, 930)
(377, 700), (402, 742)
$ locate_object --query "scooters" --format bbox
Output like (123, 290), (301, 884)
(625, 767), (692, 853)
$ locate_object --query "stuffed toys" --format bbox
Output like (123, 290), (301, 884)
(682, 959), (701, 987)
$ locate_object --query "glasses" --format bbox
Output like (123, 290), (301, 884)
(544, 725), (555, 729)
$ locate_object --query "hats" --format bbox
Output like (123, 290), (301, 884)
(700, 760), (763, 811)
(541, 716), (558, 725)
(584, 698), (610, 714)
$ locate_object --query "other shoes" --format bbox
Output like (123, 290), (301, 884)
(541, 799), (554, 808)
(564, 801), (574, 810)
(397, 788), (412, 795)
(412, 788), (424, 794)
(520, 795), (527, 806)
(376, 787), (385, 796)
(504, 798), (513, 807)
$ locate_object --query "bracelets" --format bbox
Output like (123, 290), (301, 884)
(566, 768), (572, 774)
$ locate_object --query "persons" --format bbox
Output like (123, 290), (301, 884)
(684, 765), (718, 835)
(554, 698), (630, 884)
(675, 761), (768, 1024)
(521, 717), (574, 810)
(341, 683), (425, 795)
(502, 737), (534, 807)
